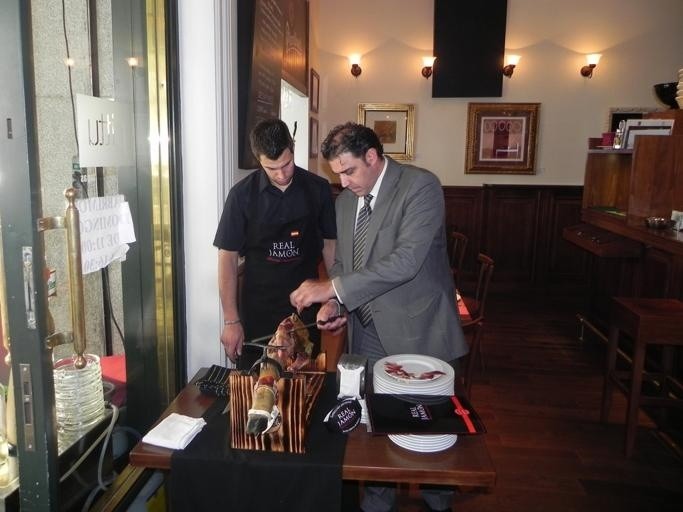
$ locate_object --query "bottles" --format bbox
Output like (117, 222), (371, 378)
(613, 129), (623, 150)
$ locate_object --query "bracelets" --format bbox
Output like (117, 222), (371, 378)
(223, 319), (241, 325)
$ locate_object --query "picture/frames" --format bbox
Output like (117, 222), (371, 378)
(464, 102), (540, 175)
(311, 68), (320, 113)
(357, 104), (416, 161)
(309, 116), (319, 158)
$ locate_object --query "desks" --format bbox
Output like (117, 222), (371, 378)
(601, 297), (683, 457)
(130, 366), (496, 512)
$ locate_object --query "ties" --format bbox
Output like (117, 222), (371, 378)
(351, 193), (373, 329)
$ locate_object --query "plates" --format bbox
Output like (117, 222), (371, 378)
(373, 355), (457, 395)
(595, 145), (614, 151)
(388, 433), (459, 453)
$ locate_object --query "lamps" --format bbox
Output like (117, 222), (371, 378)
(350, 54), (602, 80)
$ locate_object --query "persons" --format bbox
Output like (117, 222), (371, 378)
(290, 124), (472, 511)
(213, 117), (347, 375)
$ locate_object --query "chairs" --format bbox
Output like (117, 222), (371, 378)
(448, 231), (496, 398)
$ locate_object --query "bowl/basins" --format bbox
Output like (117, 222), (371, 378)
(644, 217), (677, 231)
(654, 81), (679, 109)
(675, 68), (683, 110)
(52, 355), (107, 433)
